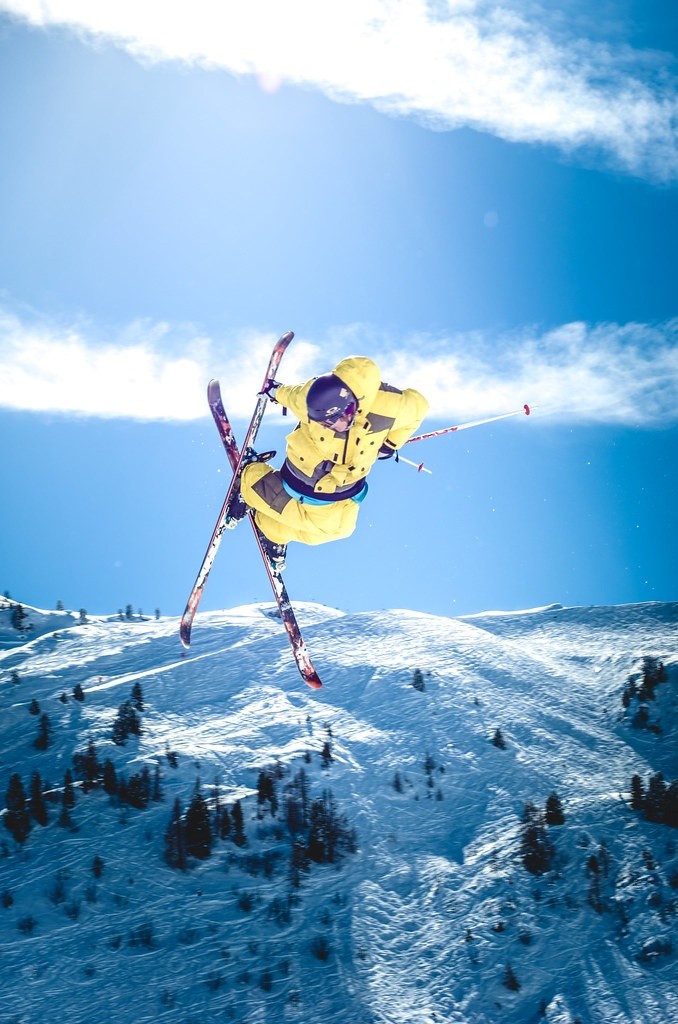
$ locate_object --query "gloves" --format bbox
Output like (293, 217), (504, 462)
(257, 379), (283, 404)
(377, 444), (395, 460)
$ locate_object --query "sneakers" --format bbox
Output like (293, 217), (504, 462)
(265, 539), (287, 562)
(230, 482), (249, 521)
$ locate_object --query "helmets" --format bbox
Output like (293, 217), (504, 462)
(307, 374), (356, 422)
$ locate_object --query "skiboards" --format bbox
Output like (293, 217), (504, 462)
(175, 327), (328, 692)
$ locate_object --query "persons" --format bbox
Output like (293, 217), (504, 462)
(225, 357), (428, 568)
